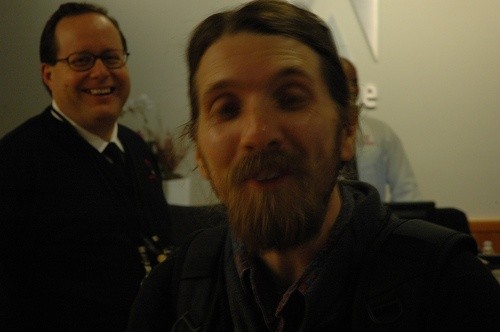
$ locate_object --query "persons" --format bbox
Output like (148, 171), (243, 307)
(125, 1), (500, 332)
(0, 0), (178, 332)
(336, 59), (423, 202)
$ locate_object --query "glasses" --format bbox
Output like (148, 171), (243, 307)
(54, 50), (130, 72)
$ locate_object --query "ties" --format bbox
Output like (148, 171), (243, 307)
(341, 154), (359, 182)
(103, 143), (140, 212)
